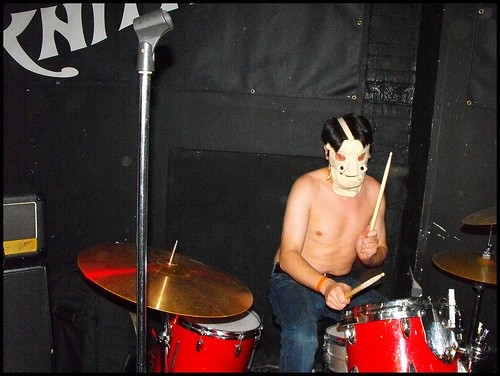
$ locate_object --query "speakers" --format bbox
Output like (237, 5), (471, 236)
(0, 259), (56, 376)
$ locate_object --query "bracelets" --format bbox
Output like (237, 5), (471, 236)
(315, 276), (327, 292)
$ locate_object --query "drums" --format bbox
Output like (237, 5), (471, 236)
(341, 297), (468, 372)
(324, 324), (349, 372)
(152, 310), (262, 373)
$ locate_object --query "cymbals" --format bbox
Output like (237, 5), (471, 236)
(77, 243), (253, 318)
(462, 206), (497, 225)
(432, 243), (497, 283)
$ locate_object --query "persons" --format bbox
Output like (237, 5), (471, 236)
(265, 113), (388, 373)
